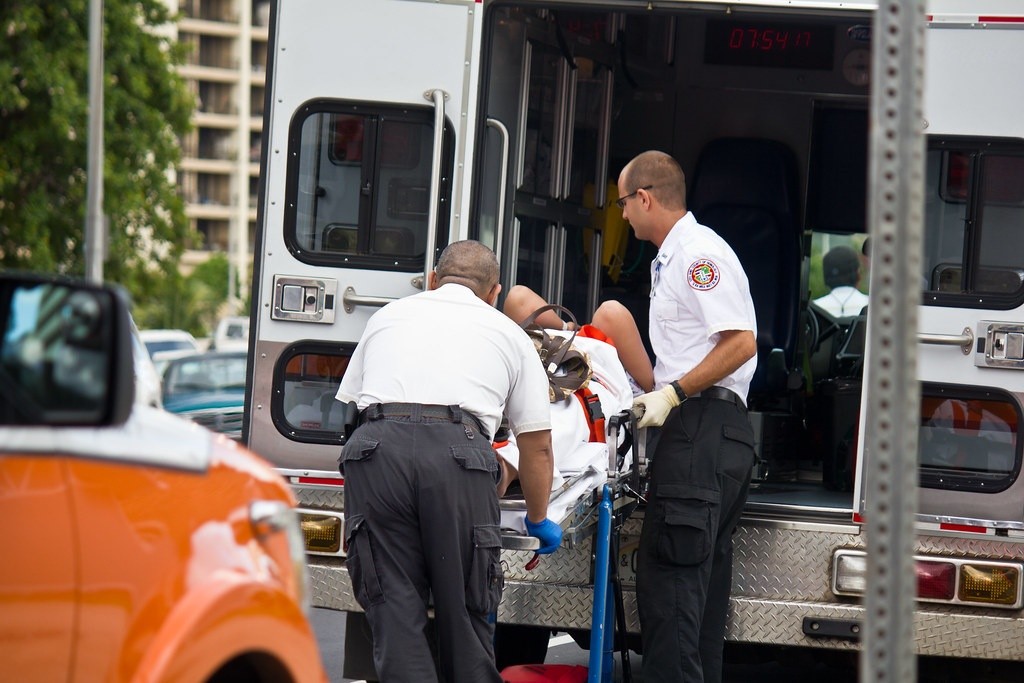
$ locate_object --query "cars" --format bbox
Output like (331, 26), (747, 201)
(139, 316), (255, 445)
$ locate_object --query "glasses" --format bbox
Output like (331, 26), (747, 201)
(616, 185), (653, 208)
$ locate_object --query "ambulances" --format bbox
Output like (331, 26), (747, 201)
(239, 0), (1021, 682)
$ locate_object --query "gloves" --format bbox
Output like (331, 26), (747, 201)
(632, 380), (688, 429)
(524, 514), (564, 554)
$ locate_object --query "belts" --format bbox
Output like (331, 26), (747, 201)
(357, 402), (480, 432)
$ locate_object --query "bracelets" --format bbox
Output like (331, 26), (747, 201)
(670, 380), (689, 404)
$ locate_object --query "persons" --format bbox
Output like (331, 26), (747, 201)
(616, 149), (761, 680)
(811, 246), (871, 316)
(336, 237), (565, 682)
(492, 283), (658, 495)
